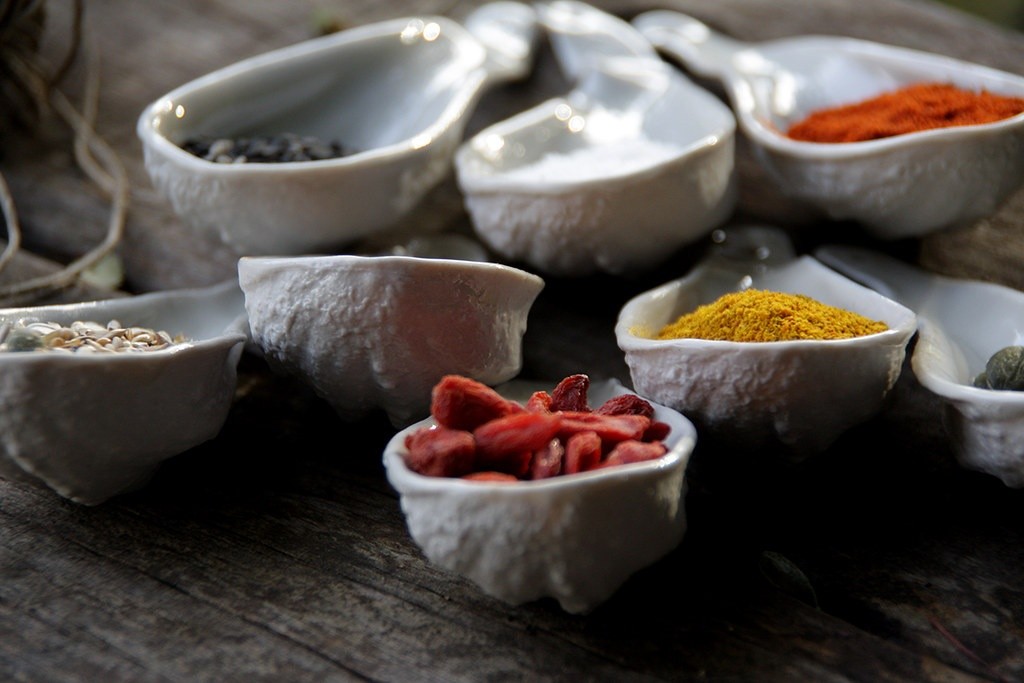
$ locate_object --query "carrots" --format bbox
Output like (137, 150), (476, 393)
(785, 79), (1024, 143)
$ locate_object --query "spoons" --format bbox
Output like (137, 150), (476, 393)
(629, 7), (1024, 238)
(814, 244), (1024, 486)
(133, 1), (537, 257)
(237, 237), (544, 427)
(615, 222), (917, 447)
(0, 276), (248, 506)
(380, 362), (697, 615)
(454, 1), (735, 269)
